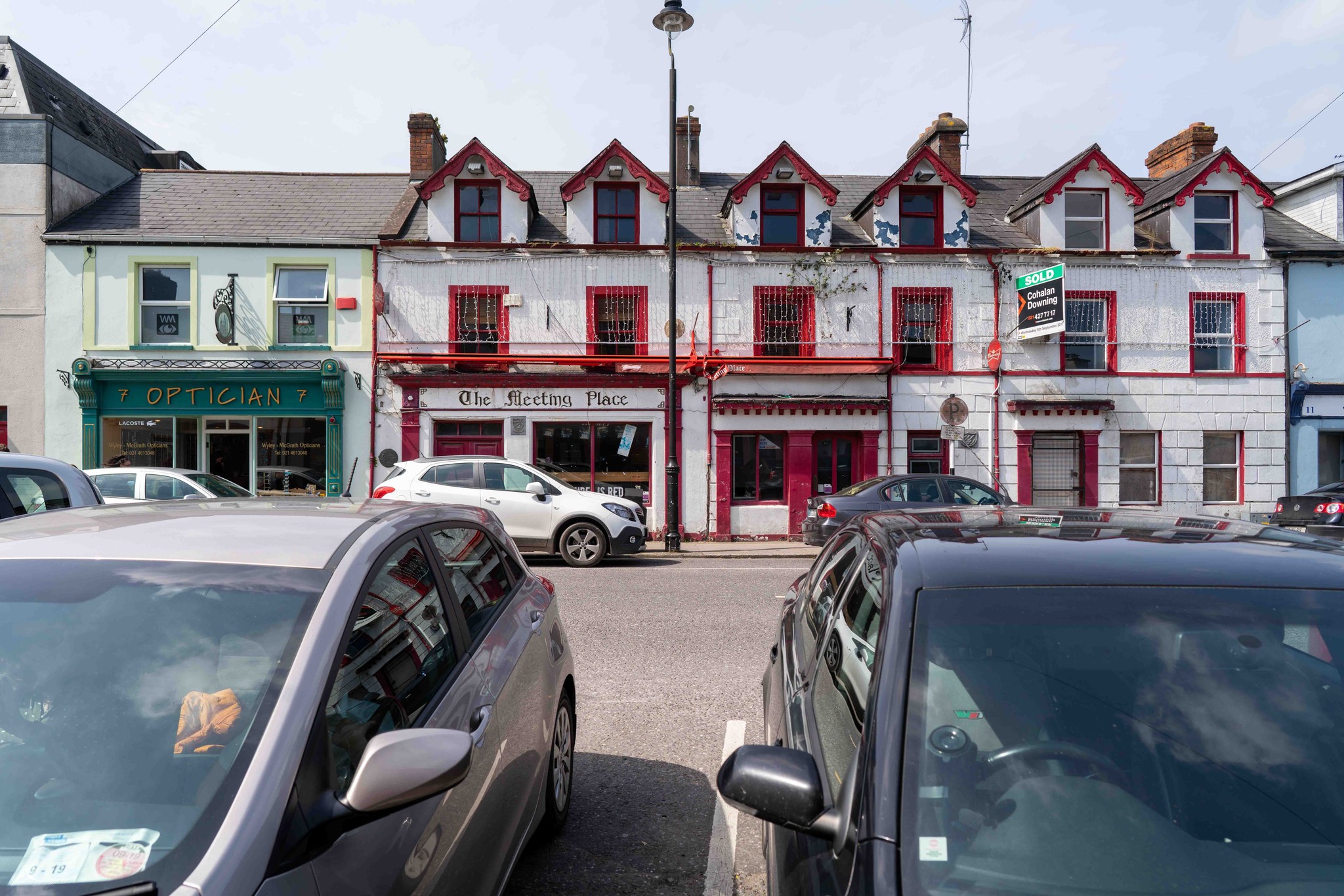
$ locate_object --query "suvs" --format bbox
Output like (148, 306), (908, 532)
(371, 454), (650, 568)
(714, 494), (1344, 896)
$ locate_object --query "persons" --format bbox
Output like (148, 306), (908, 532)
(101, 455), (131, 467)
(0, 443), (10, 452)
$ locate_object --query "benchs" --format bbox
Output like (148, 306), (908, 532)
(96, 482), (134, 499)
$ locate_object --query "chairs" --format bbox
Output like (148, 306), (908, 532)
(907, 486), (940, 503)
(0, 597), (298, 751)
(157, 486), (173, 500)
(270, 488), (326, 496)
(935, 621), (1344, 835)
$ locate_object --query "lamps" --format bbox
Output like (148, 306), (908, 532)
(768, 193), (783, 201)
(776, 167), (794, 179)
(914, 170), (936, 182)
(608, 165), (623, 177)
(608, 189), (621, 192)
(1293, 363), (1309, 378)
(903, 195), (915, 202)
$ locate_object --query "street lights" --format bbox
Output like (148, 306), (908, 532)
(651, 0), (695, 554)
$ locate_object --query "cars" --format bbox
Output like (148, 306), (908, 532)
(256, 465), (327, 496)
(1269, 478), (1344, 542)
(0, 451), (258, 522)
(0, 492), (577, 896)
(801, 473), (1026, 547)
